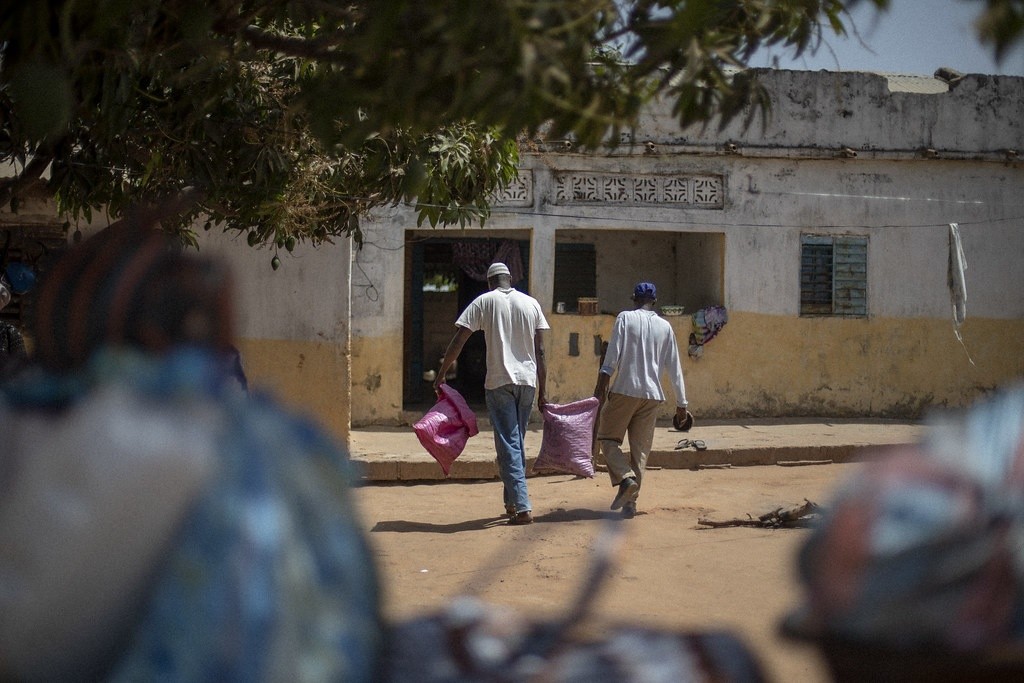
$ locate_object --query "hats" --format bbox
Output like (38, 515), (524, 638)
(487, 262), (510, 278)
(635, 283), (656, 298)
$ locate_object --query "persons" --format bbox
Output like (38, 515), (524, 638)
(594, 284), (688, 519)
(432, 263), (550, 524)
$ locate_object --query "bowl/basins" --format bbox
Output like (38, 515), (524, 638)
(579, 298), (599, 315)
(661, 306), (684, 315)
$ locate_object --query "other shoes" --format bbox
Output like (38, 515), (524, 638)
(504, 505), (516, 516)
(611, 478), (637, 510)
(621, 502), (636, 515)
(510, 511), (534, 523)
